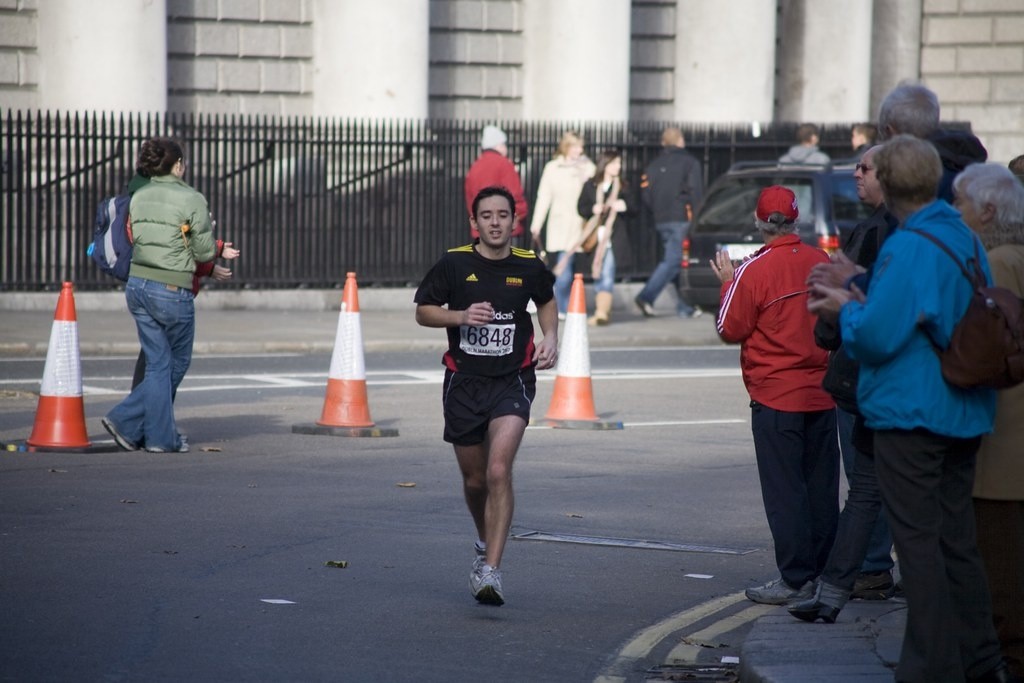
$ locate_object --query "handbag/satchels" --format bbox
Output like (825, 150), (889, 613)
(581, 218), (601, 252)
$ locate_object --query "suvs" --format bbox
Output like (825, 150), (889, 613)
(679, 156), (879, 312)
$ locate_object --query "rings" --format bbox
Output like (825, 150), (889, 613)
(718, 267), (722, 271)
(550, 360), (554, 362)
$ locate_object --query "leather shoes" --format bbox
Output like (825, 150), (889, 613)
(787, 599), (841, 624)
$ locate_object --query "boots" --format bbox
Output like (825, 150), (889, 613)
(587, 290), (614, 326)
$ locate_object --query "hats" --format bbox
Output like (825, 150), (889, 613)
(756, 185), (799, 223)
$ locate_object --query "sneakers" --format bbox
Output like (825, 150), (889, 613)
(473, 564), (506, 606)
(849, 571), (907, 602)
(471, 542), (488, 573)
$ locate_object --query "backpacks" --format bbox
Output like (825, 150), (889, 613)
(902, 224), (1023, 395)
(87, 191), (134, 283)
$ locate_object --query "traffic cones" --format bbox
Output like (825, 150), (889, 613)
(292, 271), (400, 436)
(528, 273), (625, 431)
(2, 280), (125, 452)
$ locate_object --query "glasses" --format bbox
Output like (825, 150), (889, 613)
(856, 164), (873, 174)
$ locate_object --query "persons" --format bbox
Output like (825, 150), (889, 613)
(772, 81), (1024, 683)
(413, 186), (559, 607)
(710, 185), (841, 607)
(101, 137), (240, 453)
(578, 148), (634, 325)
(634, 124), (704, 320)
(465, 125), (527, 247)
(529, 130), (597, 321)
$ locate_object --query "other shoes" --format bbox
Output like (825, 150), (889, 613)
(745, 580), (813, 605)
(101, 418), (136, 450)
(690, 310), (703, 318)
(179, 435), (189, 443)
(635, 296), (664, 317)
(145, 443), (191, 453)
(558, 312), (567, 322)
